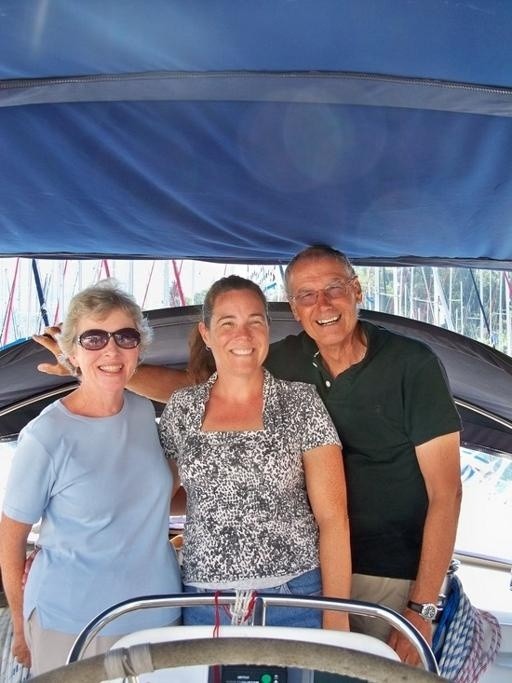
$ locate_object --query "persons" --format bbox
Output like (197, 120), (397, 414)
(19, 273), (354, 683)
(1, 276), (188, 683)
(28, 243), (465, 683)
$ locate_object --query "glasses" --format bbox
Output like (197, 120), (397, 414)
(287, 274), (358, 308)
(73, 327), (143, 351)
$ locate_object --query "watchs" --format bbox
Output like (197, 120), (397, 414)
(406, 599), (444, 622)
